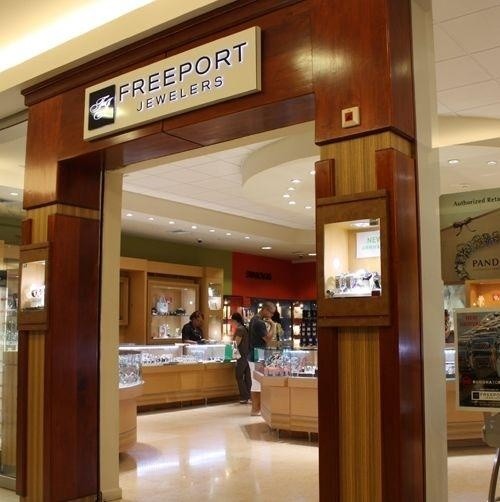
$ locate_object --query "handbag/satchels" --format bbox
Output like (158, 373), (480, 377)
(232, 341), (242, 360)
(157, 296), (168, 314)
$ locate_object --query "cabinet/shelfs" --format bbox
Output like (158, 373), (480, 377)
(147, 278), (200, 345)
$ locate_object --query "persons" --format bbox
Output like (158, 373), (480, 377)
(247, 300), (277, 416)
(231, 312), (253, 404)
(445, 308), (455, 342)
(181, 311), (210, 355)
(263, 309), (285, 342)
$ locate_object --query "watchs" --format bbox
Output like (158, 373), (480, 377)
(334, 271), (381, 292)
